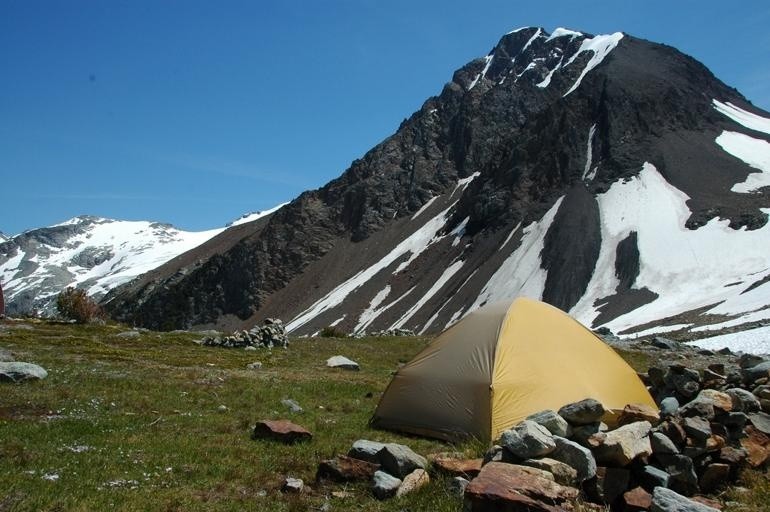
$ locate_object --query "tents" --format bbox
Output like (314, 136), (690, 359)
(371, 294), (664, 453)
